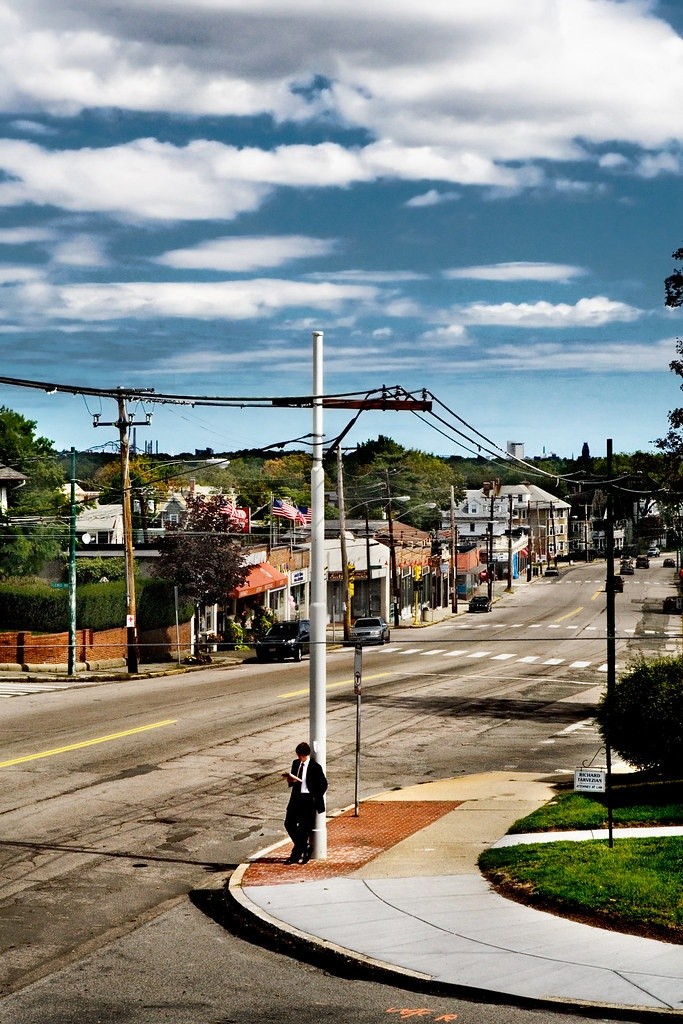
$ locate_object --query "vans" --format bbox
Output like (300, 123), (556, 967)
(636, 555), (649, 569)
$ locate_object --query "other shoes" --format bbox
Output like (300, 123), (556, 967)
(285, 857), (298, 864)
(302, 844), (312, 864)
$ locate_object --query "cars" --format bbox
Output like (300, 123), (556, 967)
(545, 566), (558, 576)
(620, 556), (633, 565)
(620, 565), (634, 575)
(479, 571), (497, 585)
(664, 558), (675, 567)
(663, 596), (683, 614)
(348, 616), (390, 646)
(469, 596), (492, 613)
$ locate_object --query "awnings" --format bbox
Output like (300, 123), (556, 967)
(521, 546), (529, 559)
(224, 563), (289, 599)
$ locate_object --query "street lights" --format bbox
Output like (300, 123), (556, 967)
(383, 467), (436, 628)
(336, 445), (409, 643)
(117, 386), (230, 675)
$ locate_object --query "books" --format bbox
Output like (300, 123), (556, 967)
(281, 772), (303, 783)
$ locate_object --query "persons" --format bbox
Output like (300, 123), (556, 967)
(283, 742), (328, 864)
(240, 602), (255, 642)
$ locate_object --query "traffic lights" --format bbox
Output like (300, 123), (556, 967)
(348, 563), (356, 583)
(414, 580), (424, 591)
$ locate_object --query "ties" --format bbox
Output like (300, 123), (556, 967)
(296, 763), (304, 793)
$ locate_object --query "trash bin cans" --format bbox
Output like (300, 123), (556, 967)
(533, 567), (539, 578)
(422, 606), (429, 622)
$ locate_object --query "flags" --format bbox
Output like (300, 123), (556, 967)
(220, 498), (239, 518)
(271, 498), (312, 528)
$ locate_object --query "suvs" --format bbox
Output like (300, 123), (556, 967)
(605, 576), (623, 593)
(254, 619), (310, 662)
(647, 548), (661, 557)
